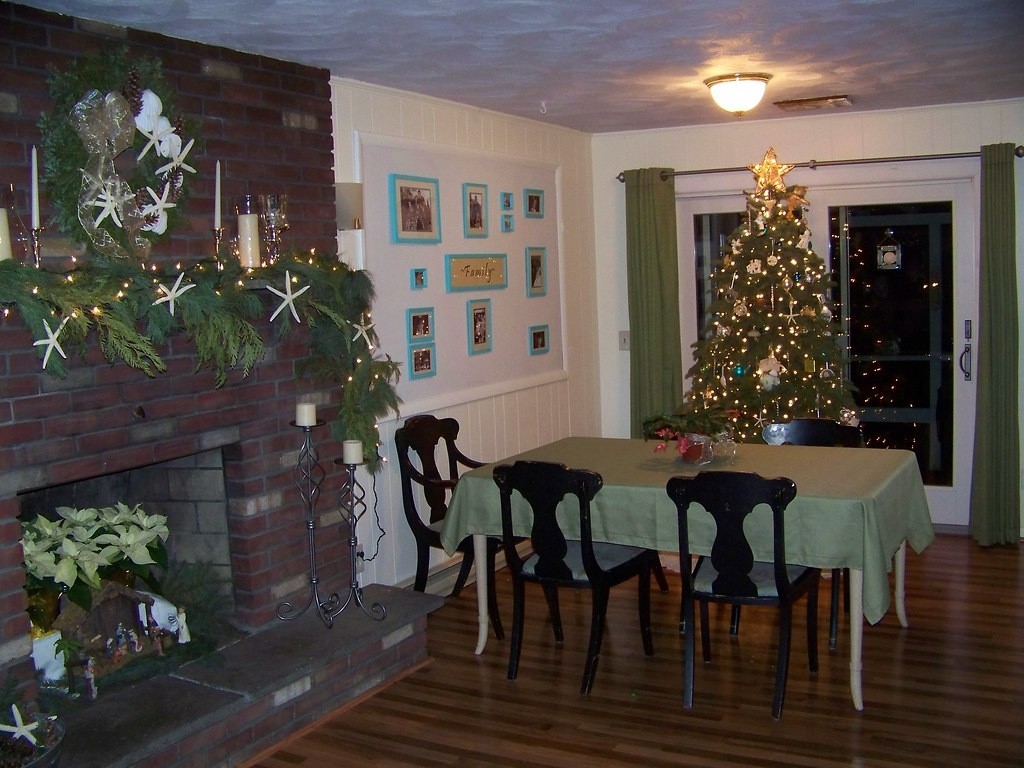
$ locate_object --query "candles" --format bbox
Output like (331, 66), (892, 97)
(237, 214), (261, 266)
(31, 145), (39, 228)
(215, 160), (220, 228)
(343, 439), (363, 464)
(296, 402), (316, 426)
(0, 208), (13, 261)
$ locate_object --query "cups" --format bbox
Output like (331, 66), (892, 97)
(683, 432), (706, 461)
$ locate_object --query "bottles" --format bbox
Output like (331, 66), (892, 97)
(237, 194), (260, 268)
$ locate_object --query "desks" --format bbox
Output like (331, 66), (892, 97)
(439, 437), (936, 712)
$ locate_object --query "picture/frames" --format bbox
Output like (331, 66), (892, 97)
(463, 182), (488, 239)
(527, 325), (550, 356)
(445, 254), (508, 292)
(525, 246), (547, 298)
(501, 192), (515, 211)
(411, 268), (428, 289)
(523, 187), (545, 219)
(467, 298), (494, 357)
(408, 343), (437, 381)
(405, 307), (435, 343)
(389, 173), (442, 243)
(501, 214), (514, 232)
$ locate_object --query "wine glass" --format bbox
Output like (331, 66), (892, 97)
(263, 194), (287, 262)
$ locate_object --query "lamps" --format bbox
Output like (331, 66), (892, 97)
(703, 72), (773, 120)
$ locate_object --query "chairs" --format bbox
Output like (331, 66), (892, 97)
(641, 414), (726, 593)
(665, 471), (822, 719)
(729, 419), (862, 653)
(394, 414), (533, 642)
(492, 459), (654, 697)
